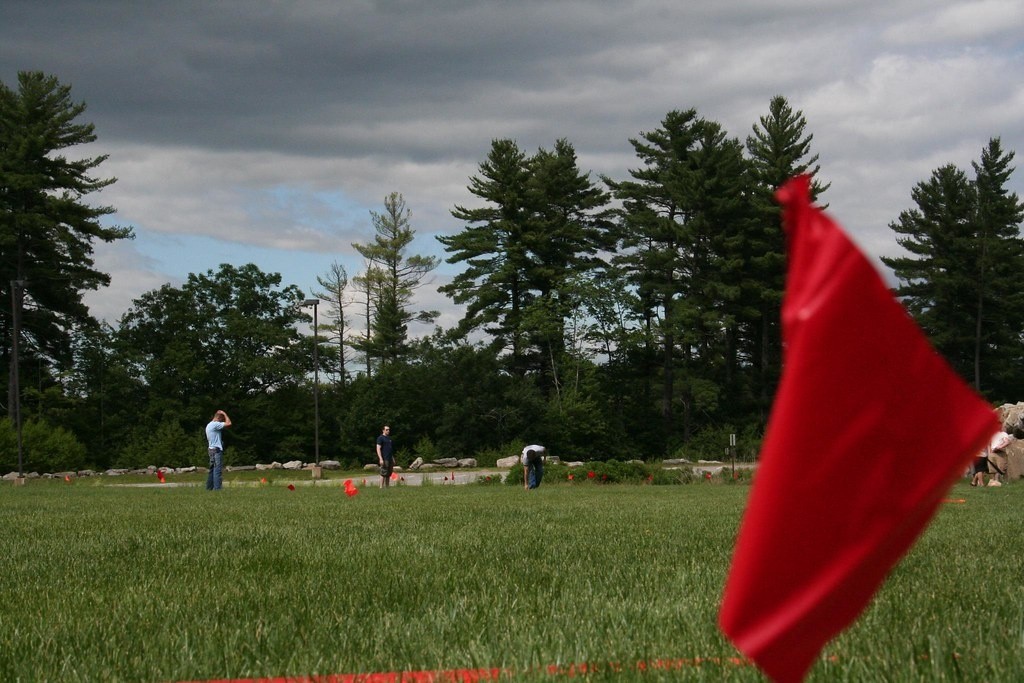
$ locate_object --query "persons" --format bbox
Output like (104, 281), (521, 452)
(969, 421), (1011, 487)
(521, 444), (546, 490)
(205, 410), (231, 490)
(376, 425), (395, 489)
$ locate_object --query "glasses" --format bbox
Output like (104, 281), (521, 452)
(386, 428), (391, 431)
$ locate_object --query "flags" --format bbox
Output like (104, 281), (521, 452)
(714, 170), (1004, 683)
